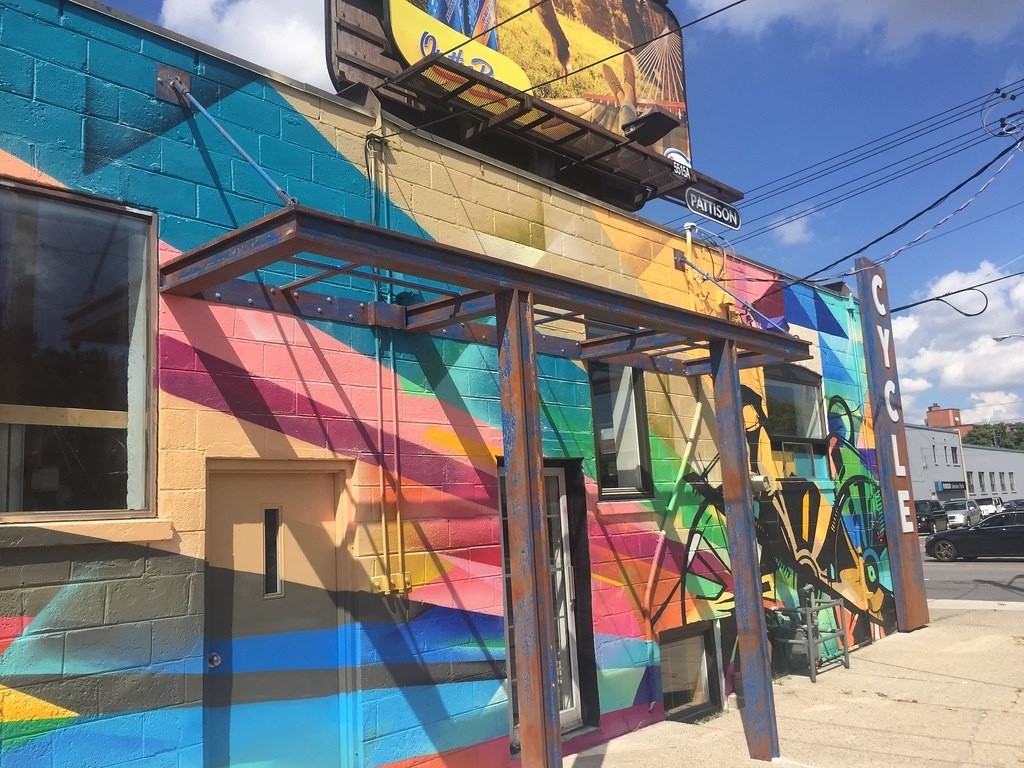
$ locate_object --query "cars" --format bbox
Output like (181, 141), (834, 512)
(925, 508), (1024, 561)
(1003, 498), (1024, 511)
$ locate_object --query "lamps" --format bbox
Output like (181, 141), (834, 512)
(558, 113), (680, 173)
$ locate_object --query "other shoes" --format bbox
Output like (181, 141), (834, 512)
(623, 54), (638, 108)
(603, 65), (625, 101)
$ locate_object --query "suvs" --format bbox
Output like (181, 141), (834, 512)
(914, 500), (950, 534)
(942, 498), (982, 528)
(976, 497), (1002, 518)
